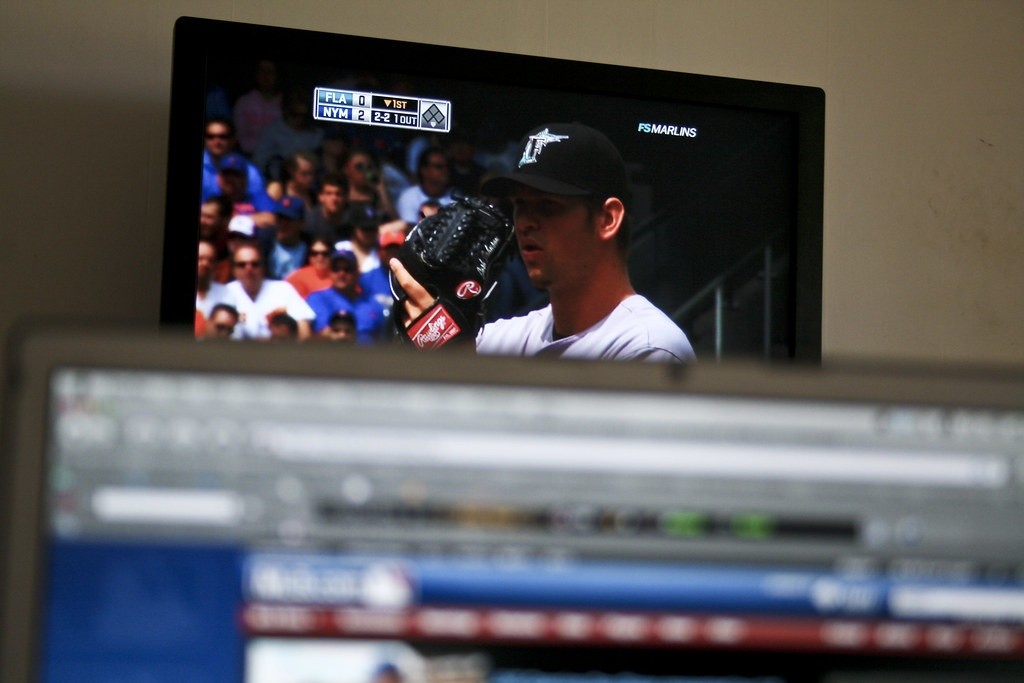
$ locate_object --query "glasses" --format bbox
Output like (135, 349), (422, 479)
(214, 322), (234, 335)
(427, 162), (449, 169)
(309, 249), (330, 257)
(331, 328), (354, 335)
(330, 264), (355, 273)
(233, 261), (263, 268)
(352, 163), (364, 170)
(205, 133), (229, 140)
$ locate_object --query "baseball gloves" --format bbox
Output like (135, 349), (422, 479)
(386, 191), (517, 355)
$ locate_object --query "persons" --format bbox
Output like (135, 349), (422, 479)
(192, 57), (540, 345)
(389, 119), (699, 366)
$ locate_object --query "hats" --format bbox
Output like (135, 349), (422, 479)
(481, 120), (625, 198)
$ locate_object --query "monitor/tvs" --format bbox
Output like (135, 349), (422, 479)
(0, 15), (1024, 683)
